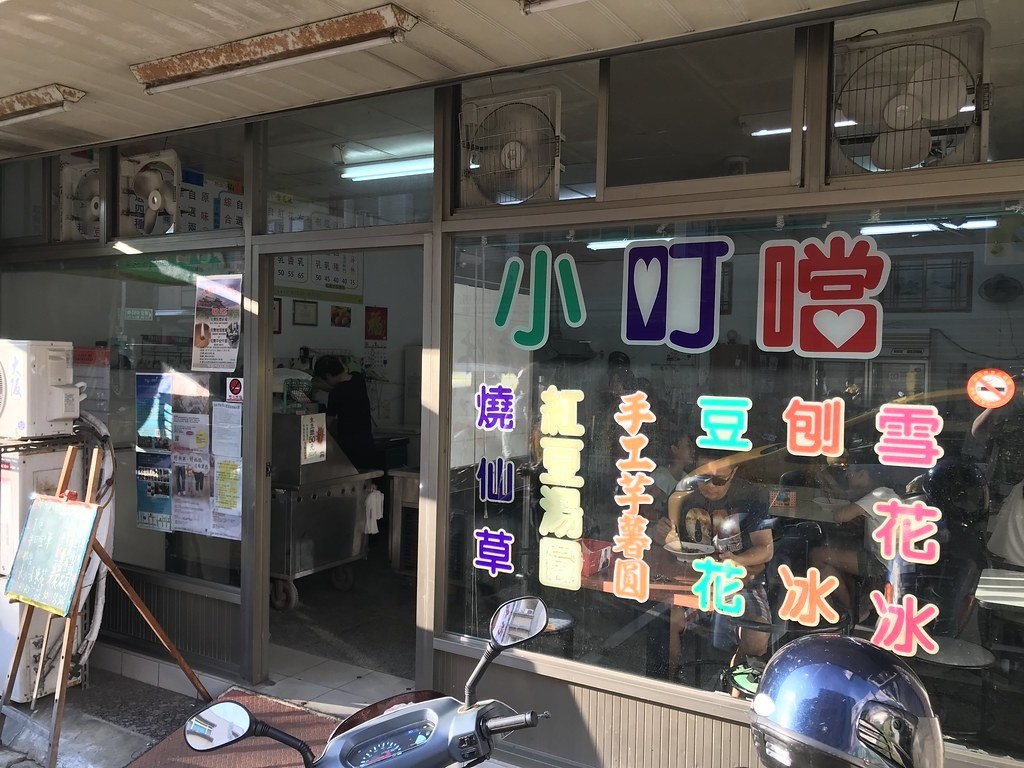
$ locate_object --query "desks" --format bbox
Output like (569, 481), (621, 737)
(974, 568), (1024, 699)
(580, 544), (765, 682)
(753, 483), (854, 546)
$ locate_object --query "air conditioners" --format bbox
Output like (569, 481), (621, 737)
(0, 340), (86, 704)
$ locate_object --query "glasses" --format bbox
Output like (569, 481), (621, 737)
(694, 464), (735, 485)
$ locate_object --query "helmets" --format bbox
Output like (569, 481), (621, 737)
(924, 461), (993, 530)
(749, 633), (944, 768)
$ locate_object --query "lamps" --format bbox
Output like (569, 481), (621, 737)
(131, 4), (419, 96)
(0, 83), (86, 127)
(340, 155), (435, 182)
(859, 219), (998, 237)
(513, 0), (589, 16)
(585, 238), (676, 251)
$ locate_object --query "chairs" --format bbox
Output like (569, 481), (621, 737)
(695, 481), (1024, 755)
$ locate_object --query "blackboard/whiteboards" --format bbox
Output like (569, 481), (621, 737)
(3, 493), (104, 617)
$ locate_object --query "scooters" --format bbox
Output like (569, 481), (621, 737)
(185, 594), (553, 768)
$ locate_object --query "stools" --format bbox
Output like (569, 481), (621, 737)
(525, 605), (576, 659)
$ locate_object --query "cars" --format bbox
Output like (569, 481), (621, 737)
(665, 386), (1024, 626)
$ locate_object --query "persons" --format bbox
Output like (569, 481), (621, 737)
(176, 463), (204, 495)
(605, 348), (1024, 700)
(314, 354), (371, 474)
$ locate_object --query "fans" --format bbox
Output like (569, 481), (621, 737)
(978, 273), (1023, 304)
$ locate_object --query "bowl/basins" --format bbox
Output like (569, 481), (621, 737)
(664, 541), (715, 563)
(812, 497), (850, 511)
